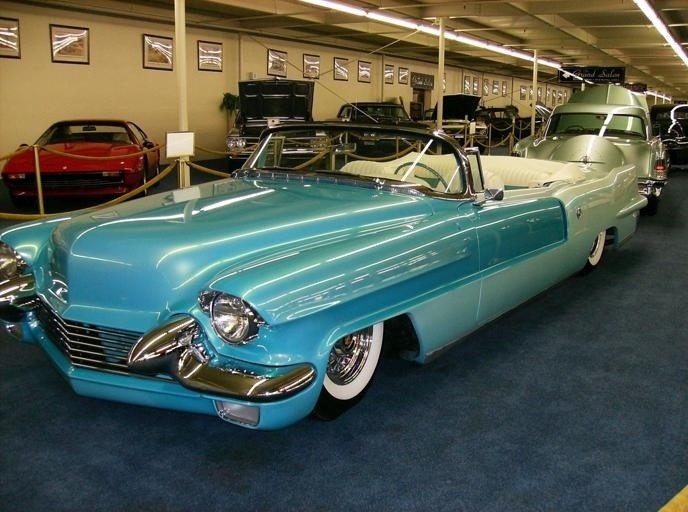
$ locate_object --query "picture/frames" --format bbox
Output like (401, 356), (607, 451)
(463, 76), (569, 107)
(49, 23), (90, 64)
(142, 34), (173, 71)
(443, 73), (447, 92)
(197, 40), (223, 72)
(267, 48), (409, 84)
(0, 17), (21, 59)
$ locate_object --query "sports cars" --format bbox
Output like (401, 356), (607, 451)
(1, 122), (650, 427)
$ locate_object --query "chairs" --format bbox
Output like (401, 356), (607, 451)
(112, 133), (130, 144)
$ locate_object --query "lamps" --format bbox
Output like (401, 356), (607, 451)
(632, 0), (688, 66)
(299, 0), (563, 70)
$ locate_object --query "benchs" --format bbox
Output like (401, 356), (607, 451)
(339, 151), (504, 196)
(466, 155), (584, 188)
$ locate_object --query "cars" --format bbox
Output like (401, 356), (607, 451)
(511, 85), (667, 212)
(429, 94), (546, 154)
(227, 79), (320, 158)
(649, 102), (687, 169)
(1, 118), (162, 207)
(339, 103), (419, 156)
